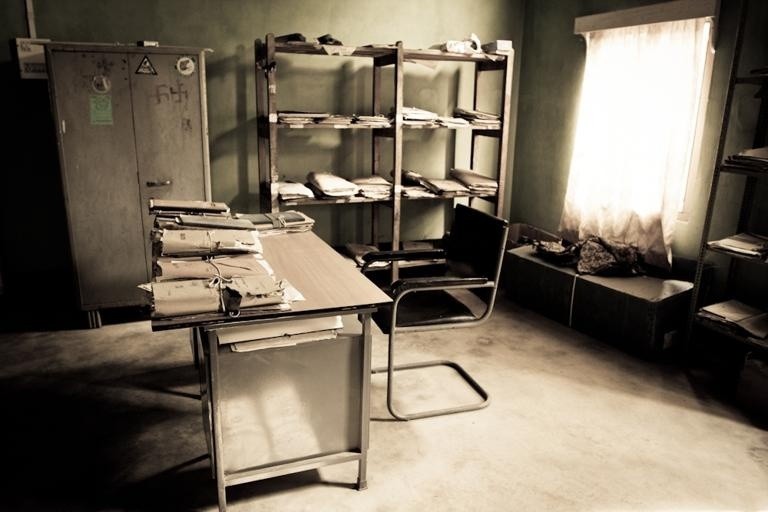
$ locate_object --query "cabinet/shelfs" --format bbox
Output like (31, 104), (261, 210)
(507, 243), (695, 362)
(252, 34), (514, 283)
(680, 21), (768, 423)
(44, 39), (213, 331)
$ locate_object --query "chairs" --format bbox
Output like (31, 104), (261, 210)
(358, 200), (510, 421)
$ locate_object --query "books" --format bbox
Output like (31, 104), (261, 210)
(277, 173), (392, 200)
(390, 167), (498, 198)
(697, 145), (768, 343)
(402, 106), (500, 126)
(277, 111), (391, 125)
(145, 200), (315, 319)
(344, 242), (437, 268)
(441, 38), (512, 56)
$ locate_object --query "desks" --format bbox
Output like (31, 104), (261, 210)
(150, 212), (395, 512)
